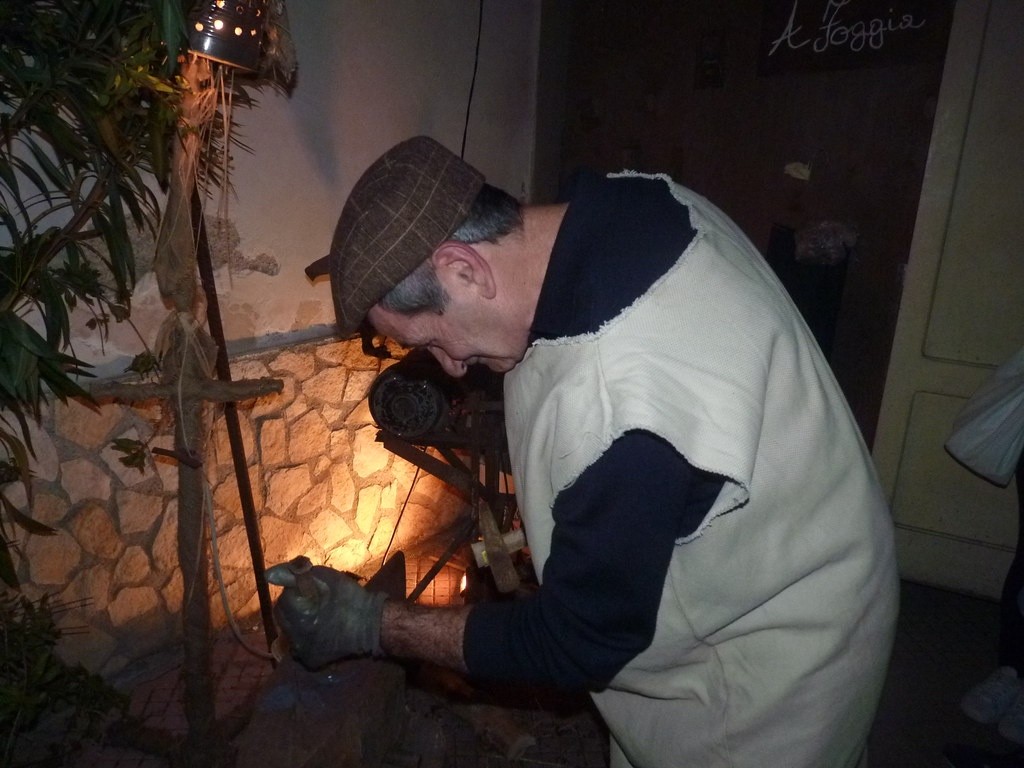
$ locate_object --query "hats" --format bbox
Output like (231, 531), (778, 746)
(328, 136), (486, 340)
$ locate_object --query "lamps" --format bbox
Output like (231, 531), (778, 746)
(186, 1), (271, 74)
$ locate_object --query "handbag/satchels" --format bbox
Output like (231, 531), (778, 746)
(944, 345), (1024, 488)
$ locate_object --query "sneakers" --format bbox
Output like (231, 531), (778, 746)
(998, 688), (1024, 744)
(961, 666), (1024, 724)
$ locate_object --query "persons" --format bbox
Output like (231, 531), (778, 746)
(265, 135), (900, 768)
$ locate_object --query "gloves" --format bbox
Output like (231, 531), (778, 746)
(263, 555), (390, 670)
